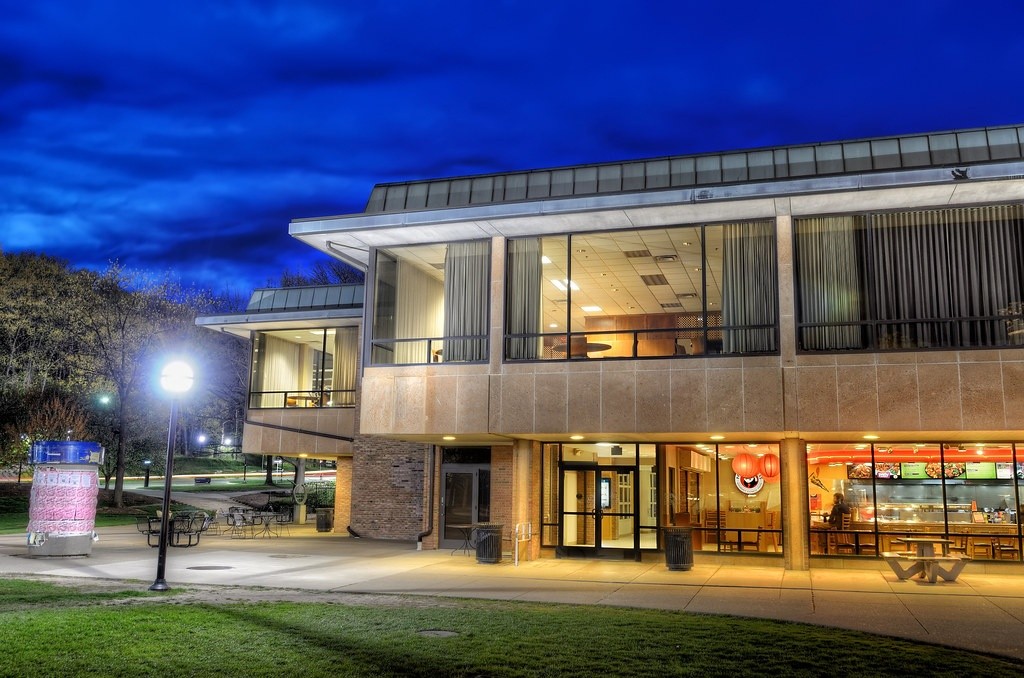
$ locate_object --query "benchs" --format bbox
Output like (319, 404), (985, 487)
(195, 477), (211, 484)
(880, 552), (972, 564)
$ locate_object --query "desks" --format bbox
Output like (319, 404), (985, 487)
(446, 524), (476, 557)
(848, 528), (871, 554)
(172, 509), (202, 530)
(251, 514), (283, 538)
(880, 538), (972, 583)
(552, 343), (611, 359)
(151, 518), (191, 546)
(894, 527), (923, 550)
(691, 338), (723, 355)
(288, 396), (318, 407)
(222, 513), (251, 539)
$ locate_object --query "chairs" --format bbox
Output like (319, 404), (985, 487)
(743, 526), (763, 551)
(970, 531), (992, 558)
(913, 527), (930, 551)
(946, 530), (970, 555)
(991, 531), (1018, 559)
(828, 514), (855, 554)
(571, 336), (591, 358)
(886, 525), (906, 551)
(691, 337), (704, 353)
(715, 525), (732, 551)
(137, 506), (294, 548)
(860, 529), (881, 555)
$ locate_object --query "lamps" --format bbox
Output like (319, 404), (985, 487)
(888, 447), (892, 454)
(958, 444), (966, 451)
(944, 444), (950, 449)
(913, 446), (918, 453)
(877, 447), (887, 452)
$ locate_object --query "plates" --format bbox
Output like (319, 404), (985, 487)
(874, 466), (900, 476)
(924, 462), (965, 478)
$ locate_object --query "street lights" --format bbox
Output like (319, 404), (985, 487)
(17, 435), (29, 483)
(148, 360), (194, 592)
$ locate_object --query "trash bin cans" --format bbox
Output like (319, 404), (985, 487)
(661, 527), (694, 572)
(314, 508), (334, 532)
(473, 522), (505, 565)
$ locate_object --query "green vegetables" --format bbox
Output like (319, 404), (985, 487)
(936, 463), (965, 478)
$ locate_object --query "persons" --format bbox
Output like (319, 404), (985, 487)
(828, 493), (850, 530)
